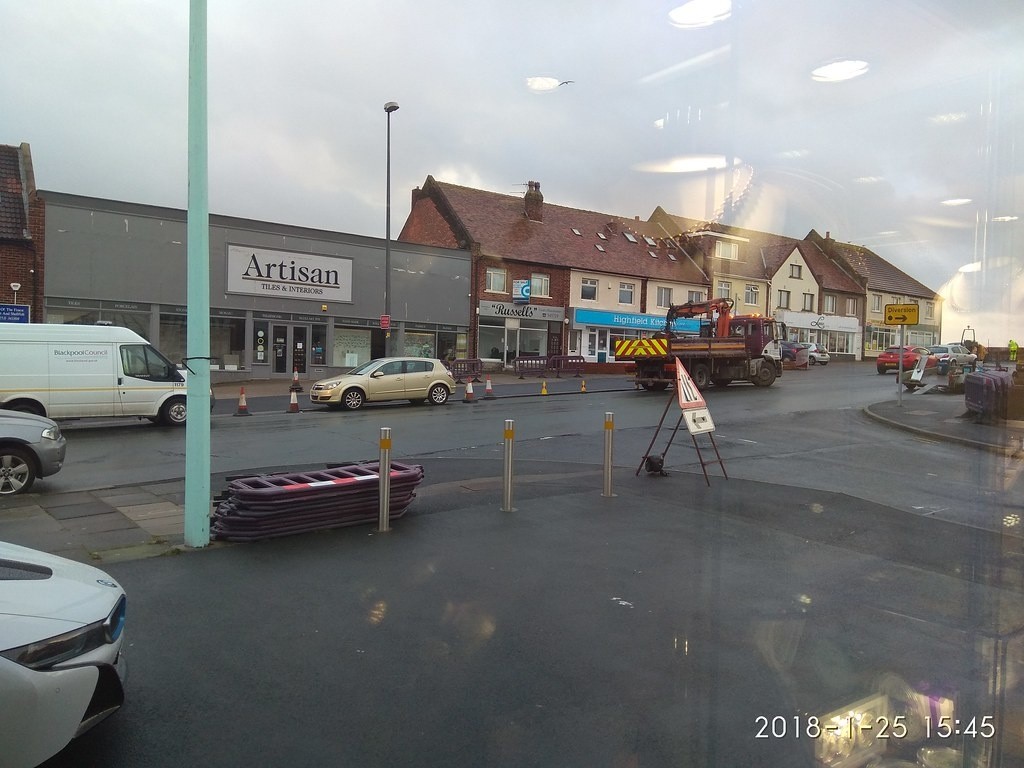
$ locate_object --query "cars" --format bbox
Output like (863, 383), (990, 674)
(0, 538), (129, 768)
(876, 345), (939, 374)
(0, 409), (67, 497)
(310, 357), (456, 411)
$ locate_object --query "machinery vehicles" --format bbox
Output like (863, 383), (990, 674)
(614, 297), (788, 391)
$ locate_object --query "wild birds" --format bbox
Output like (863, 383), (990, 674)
(559, 81), (575, 86)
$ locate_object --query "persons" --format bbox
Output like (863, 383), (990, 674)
(967, 344), (977, 373)
(1008, 340), (1018, 361)
(977, 344), (985, 361)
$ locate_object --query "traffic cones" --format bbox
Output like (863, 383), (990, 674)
(288, 366), (305, 393)
(483, 373), (498, 400)
(232, 386), (252, 417)
(462, 376), (478, 403)
(286, 386), (301, 414)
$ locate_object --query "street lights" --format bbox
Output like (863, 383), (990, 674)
(10, 282), (21, 304)
(383, 100), (400, 356)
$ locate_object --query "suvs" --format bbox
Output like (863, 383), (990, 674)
(927, 344), (978, 374)
(782, 344), (805, 363)
(798, 342), (831, 365)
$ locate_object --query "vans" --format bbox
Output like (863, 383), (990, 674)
(0, 323), (217, 426)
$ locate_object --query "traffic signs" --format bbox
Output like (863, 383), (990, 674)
(884, 303), (920, 325)
